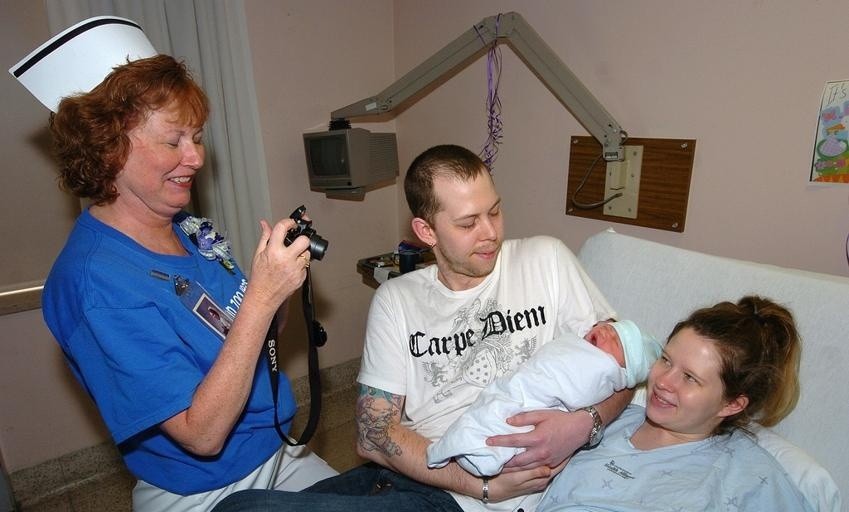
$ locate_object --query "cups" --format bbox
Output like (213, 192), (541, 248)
(393, 251), (419, 273)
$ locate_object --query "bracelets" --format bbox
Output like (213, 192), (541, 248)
(482, 478), (489, 504)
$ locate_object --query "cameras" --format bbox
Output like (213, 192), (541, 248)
(266, 205), (331, 262)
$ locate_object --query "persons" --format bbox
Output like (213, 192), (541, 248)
(530, 294), (812, 512)
(210, 147), (637, 512)
(8, 16), (339, 512)
(207, 307), (229, 333)
(582, 320), (626, 367)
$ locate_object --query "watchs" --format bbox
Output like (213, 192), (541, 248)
(574, 406), (604, 450)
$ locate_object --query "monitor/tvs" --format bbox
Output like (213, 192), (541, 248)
(301, 128), (400, 202)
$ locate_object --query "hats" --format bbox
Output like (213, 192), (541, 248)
(611, 319), (663, 385)
(8, 16), (158, 115)
